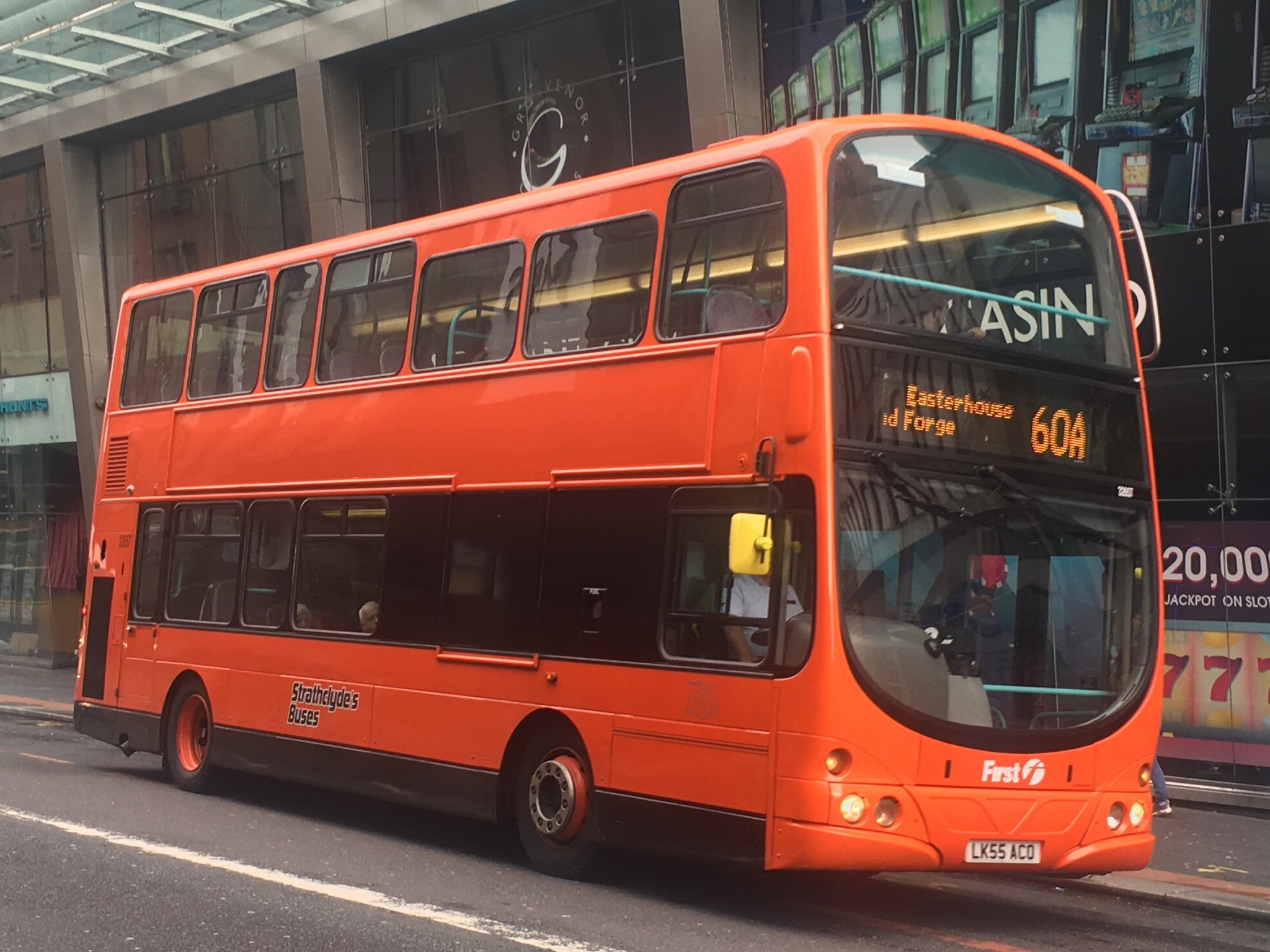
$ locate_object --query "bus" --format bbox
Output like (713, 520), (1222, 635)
(71, 114), (1165, 883)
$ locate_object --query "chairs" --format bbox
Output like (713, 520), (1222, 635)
(199, 575), (330, 627)
(137, 281), (789, 404)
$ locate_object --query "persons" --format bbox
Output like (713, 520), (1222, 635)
(295, 603), (311, 629)
(1151, 757), (1171, 815)
(903, 295), (984, 337)
(721, 564), (805, 662)
(358, 602), (379, 633)
